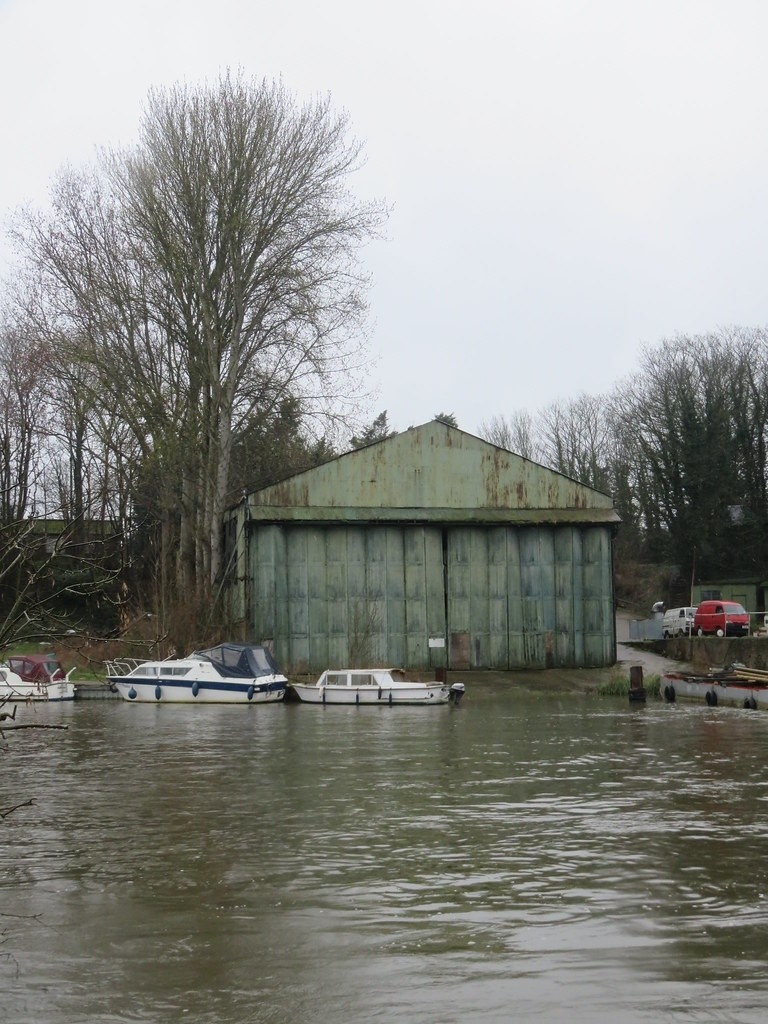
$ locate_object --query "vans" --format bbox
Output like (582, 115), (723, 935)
(695, 601), (751, 636)
(661, 607), (698, 636)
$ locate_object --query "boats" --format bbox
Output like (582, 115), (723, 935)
(657, 672), (768, 709)
(291, 667), (465, 704)
(105, 644), (291, 705)
(0, 655), (77, 702)
